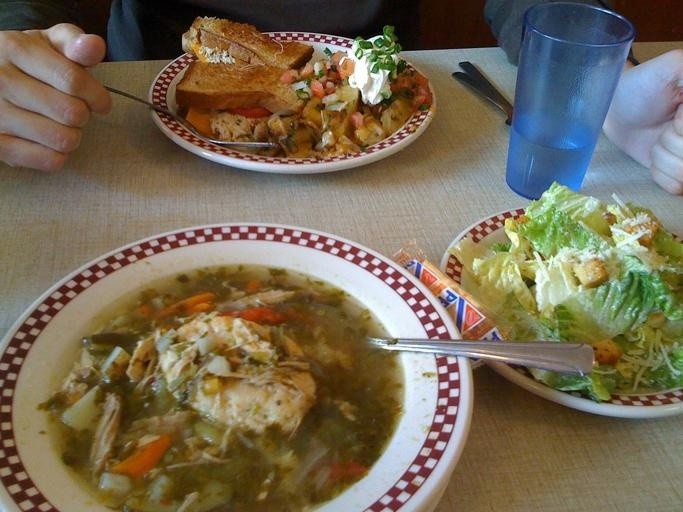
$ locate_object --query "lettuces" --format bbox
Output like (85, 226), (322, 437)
(450, 181), (682, 403)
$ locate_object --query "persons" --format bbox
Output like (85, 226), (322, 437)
(0, 0), (682, 198)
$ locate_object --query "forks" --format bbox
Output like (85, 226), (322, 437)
(99, 84), (282, 147)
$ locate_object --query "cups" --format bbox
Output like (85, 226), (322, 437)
(505, 1), (635, 204)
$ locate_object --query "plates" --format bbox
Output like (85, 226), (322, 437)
(438, 205), (682, 422)
(145, 29), (436, 175)
(0, 219), (471, 512)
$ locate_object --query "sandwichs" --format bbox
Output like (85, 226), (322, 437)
(173, 16), (314, 158)
